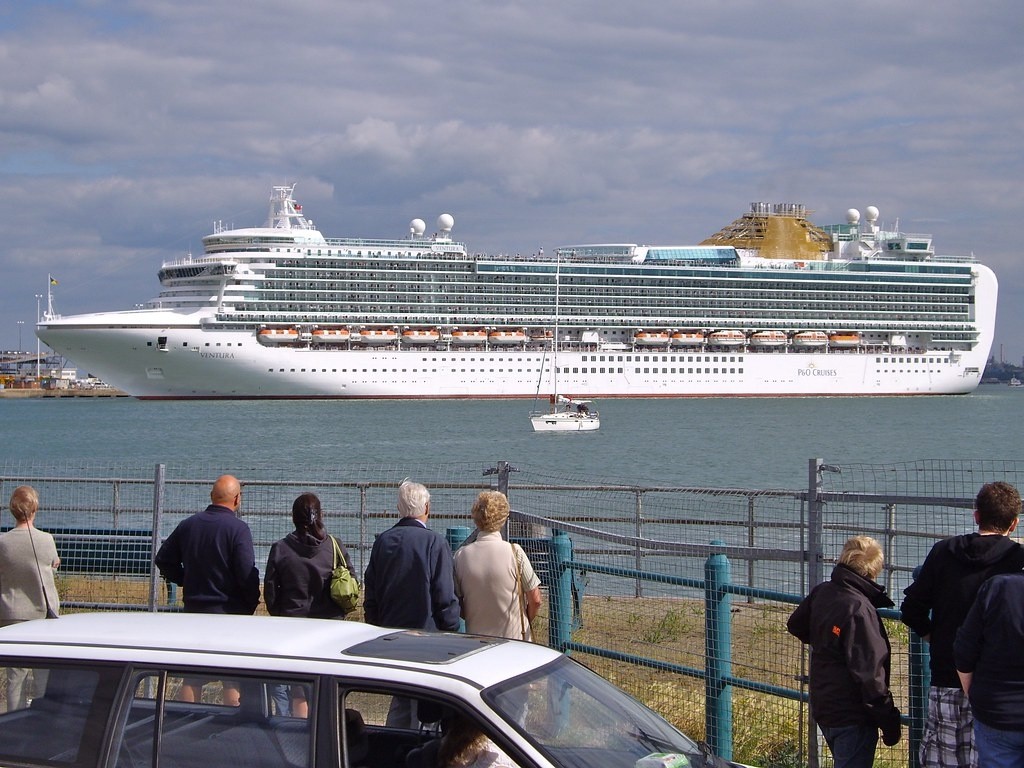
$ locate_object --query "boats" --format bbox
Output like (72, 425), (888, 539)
(1008, 373), (1023, 387)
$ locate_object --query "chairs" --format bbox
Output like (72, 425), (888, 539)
(166, 709), (373, 768)
(405, 698), (447, 768)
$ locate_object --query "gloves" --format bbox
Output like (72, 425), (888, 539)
(880, 707), (901, 746)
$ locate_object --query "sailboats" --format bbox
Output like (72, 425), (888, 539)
(530, 252), (600, 431)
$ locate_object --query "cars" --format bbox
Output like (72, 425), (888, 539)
(0, 612), (754, 768)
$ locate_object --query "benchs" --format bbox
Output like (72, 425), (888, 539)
(0, 527), (177, 605)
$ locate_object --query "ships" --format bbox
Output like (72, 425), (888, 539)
(35, 182), (998, 398)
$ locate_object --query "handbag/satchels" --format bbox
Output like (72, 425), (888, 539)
(328, 534), (360, 613)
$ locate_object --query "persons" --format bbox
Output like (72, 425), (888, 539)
(362, 481), (461, 731)
(153, 474), (262, 706)
(453, 490), (542, 730)
(261, 493), (360, 719)
(900, 480), (1024, 768)
(785, 535), (902, 768)
(0, 484), (62, 712)
(404, 697), (520, 768)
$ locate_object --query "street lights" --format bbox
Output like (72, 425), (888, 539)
(34, 294), (42, 381)
(17, 320), (24, 352)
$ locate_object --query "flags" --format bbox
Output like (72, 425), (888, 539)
(50, 276), (59, 286)
